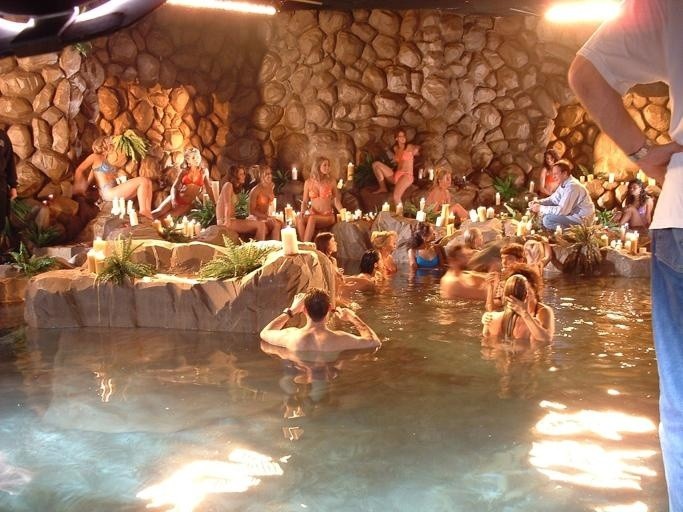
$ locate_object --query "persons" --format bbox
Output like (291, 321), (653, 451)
(567, 0), (683, 512)
(73, 129), (653, 362)
(0, 128), (19, 232)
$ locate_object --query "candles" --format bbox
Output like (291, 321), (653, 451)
(83, 165), (660, 277)
